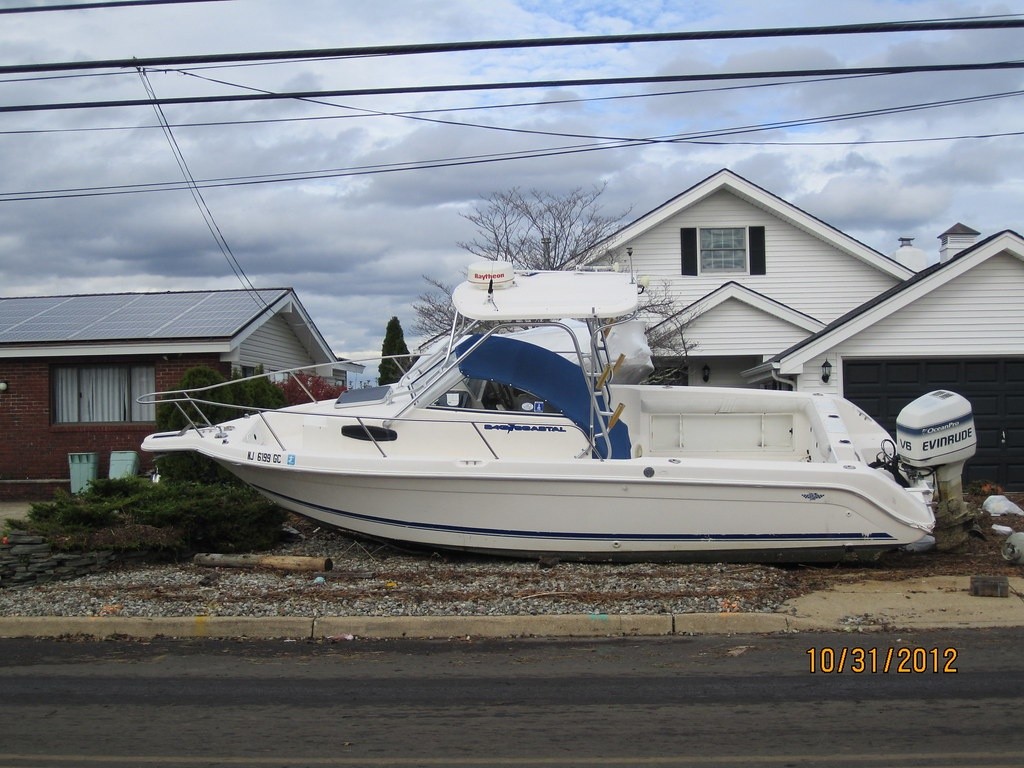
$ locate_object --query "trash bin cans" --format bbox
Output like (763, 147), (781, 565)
(68, 451), (100, 494)
(109, 450), (142, 480)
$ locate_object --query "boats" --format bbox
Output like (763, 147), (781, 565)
(133, 261), (979, 562)
